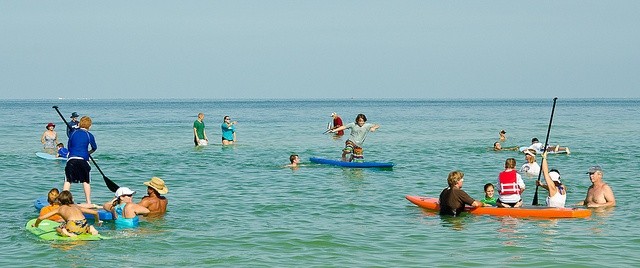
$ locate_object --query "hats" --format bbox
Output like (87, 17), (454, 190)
(586, 166), (601, 174)
(115, 187), (135, 198)
(549, 171), (561, 184)
(144, 176), (168, 195)
(70, 112), (79, 118)
(46, 123), (55, 130)
(523, 149), (536, 157)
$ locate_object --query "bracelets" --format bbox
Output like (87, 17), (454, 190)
(540, 155), (550, 160)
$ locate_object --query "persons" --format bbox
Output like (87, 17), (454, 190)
(55, 142), (69, 158)
(104, 187), (149, 232)
(327, 113), (344, 134)
(332, 114), (381, 162)
(40, 188), (63, 223)
(62, 116), (96, 207)
(537, 150), (566, 208)
(480, 183), (497, 205)
(499, 130), (510, 141)
(34, 191), (103, 236)
(438, 170), (483, 219)
(41, 123), (58, 150)
(496, 158), (525, 208)
(193, 112), (208, 146)
(66, 111), (81, 137)
(523, 138), (570, 154)
(135, 177), (168, 216)
(571, 166), (616, 212)
(284, 154), (306, 168)
(492, 141), (502, 150)
(221, 116), (236, 145)
(519, 149), (540, 175)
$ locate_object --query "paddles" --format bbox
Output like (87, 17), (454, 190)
(532, 97), (558, 205)
(52, 106), (120, 192)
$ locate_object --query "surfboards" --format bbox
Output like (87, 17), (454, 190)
(34, 196), (113, 220)
(26, 219), (100, 241)
(519, 144), (571, 158)
(310, 157), (394, 168)
(405, 194), (593, 219)
(36, 152), (98, 162)
(323, 125), (341, 135)
